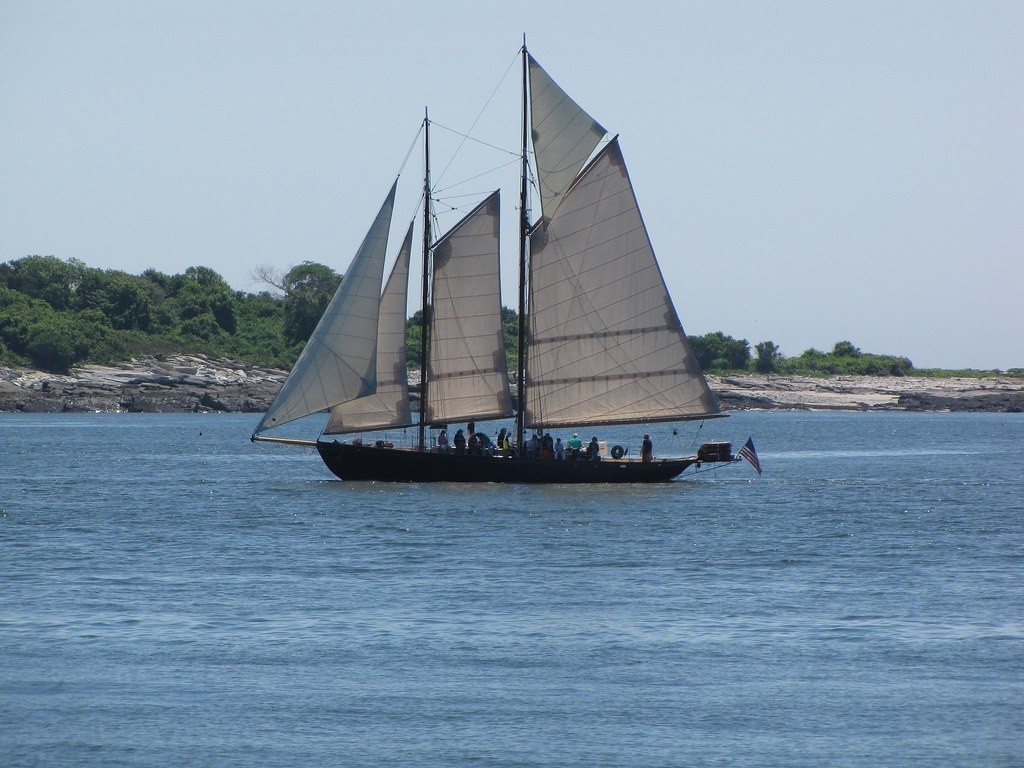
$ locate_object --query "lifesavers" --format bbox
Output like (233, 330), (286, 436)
(610, 444), (625, 459)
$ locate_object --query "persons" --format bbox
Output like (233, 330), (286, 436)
(523, 432), (553, 459)
(503, 432), (519, 457)
(438, 430), (451, 454)
(497, 427), (506, 448)
(588, 437), (599, 456)
(639, 433), (653, 462)
(466, 422), (485, 457)
(454, 429), (465, 447)
(554, 438), (566, 460)
(567, 433), (585, 455)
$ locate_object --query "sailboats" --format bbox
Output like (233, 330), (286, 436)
(247, 31), (736, 482)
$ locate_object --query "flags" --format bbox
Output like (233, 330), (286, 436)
(740, 437), (762, 475)
